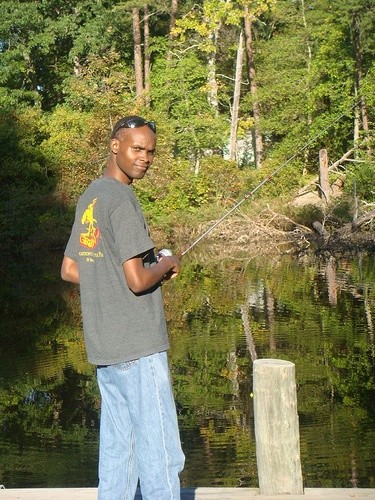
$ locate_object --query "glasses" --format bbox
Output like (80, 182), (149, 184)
(113, 120), (157, 138)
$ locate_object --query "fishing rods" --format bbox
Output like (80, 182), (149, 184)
(157, 97), (368, 264)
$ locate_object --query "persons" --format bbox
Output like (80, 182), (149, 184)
(61, 116), (187, 499)
